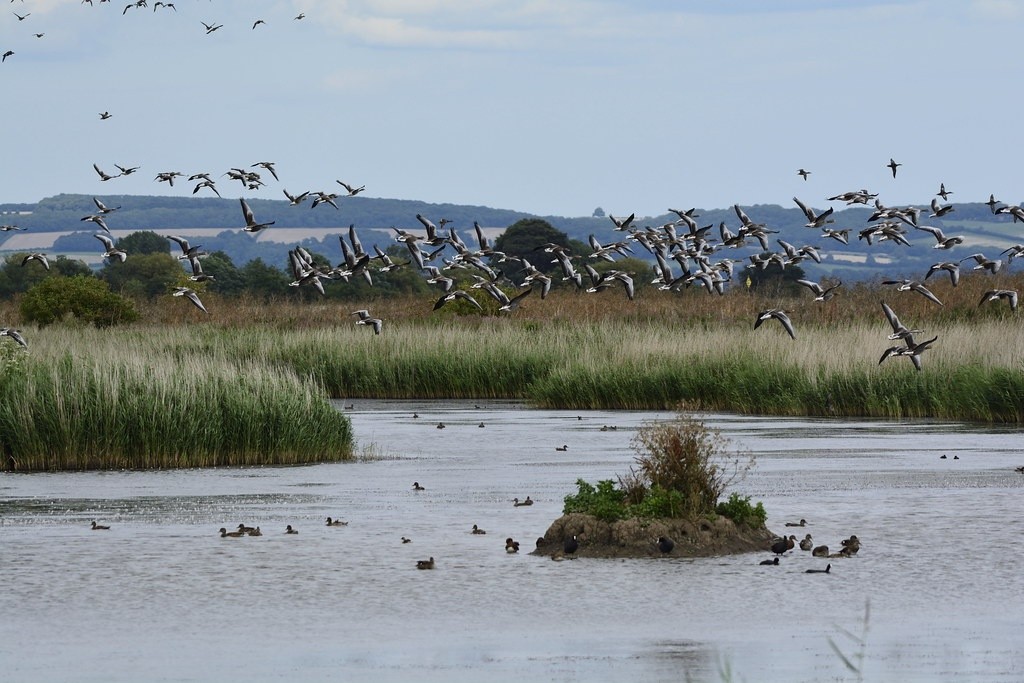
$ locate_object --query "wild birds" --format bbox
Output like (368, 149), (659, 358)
(153, 162), (279, 198)
(200, 13), (306, 35)
(344, 403), (619, 452)
(392, 214), (554, 312)
(878, 300), (938, 372)
(562, 519), (862, 575)
(80, 197), (217, 314)
(940, 454), (960, 460)
(0, 225), (50, 271)
(239, 180), (365, 233)
(288, 224), (412, 295)
(533, 205), (822, 340)
(90, 481), (533, 571)
(0, 0), (44, 63)
(793, 159), (1024, 313)
(80, 0), (176, 15)
(93, 163), (140, 181)
(0, 328), (29, 350)
(351, 310), (382, 335)
(99, 112), (112, 120)
